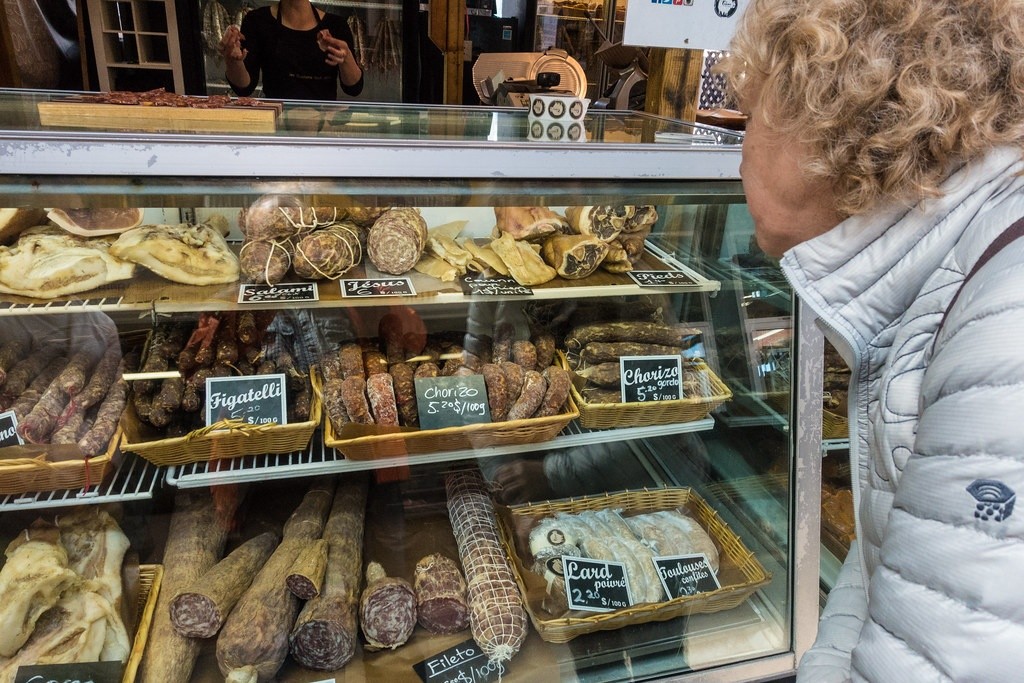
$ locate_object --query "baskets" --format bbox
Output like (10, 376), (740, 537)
(325, 349), (572, 457)
(123, 372), (314, 465)
(0, 322), (152, 496)
(107, 565), (165, 681)
(489, 482), (771, 642)
(557, 324), (730, 430)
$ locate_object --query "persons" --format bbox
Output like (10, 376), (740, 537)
(725, 1), (1022, 681)
(219, 0), (365, 101)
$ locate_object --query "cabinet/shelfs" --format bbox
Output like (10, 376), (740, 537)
(1, 86), (827, 682)
